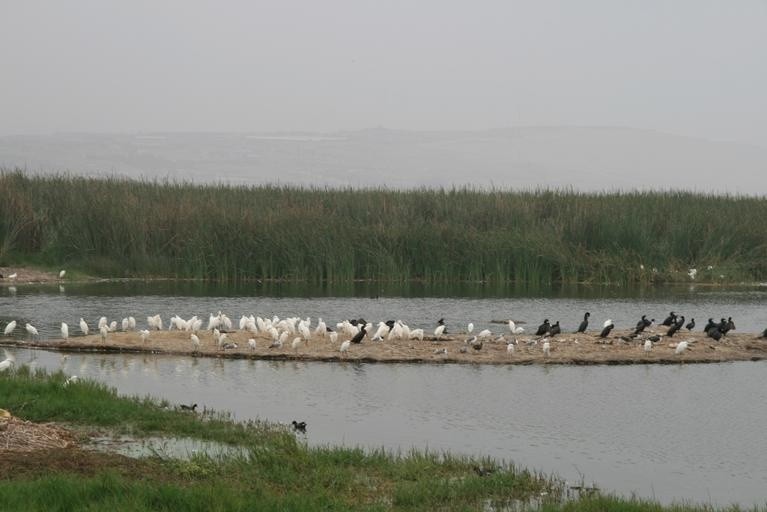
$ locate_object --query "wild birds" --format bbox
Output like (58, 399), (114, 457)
(25, 322), (39, 340)
(79, 317), (90, 335)
(180, 404), (198, 412)
(0, 358), (15, 371)
(63, 375), (78, 389)
(61, 321), (69, 340)
(4, 320), (17, 337)
(98, 311), (736, 358)
(292, 420), (307, 429)
